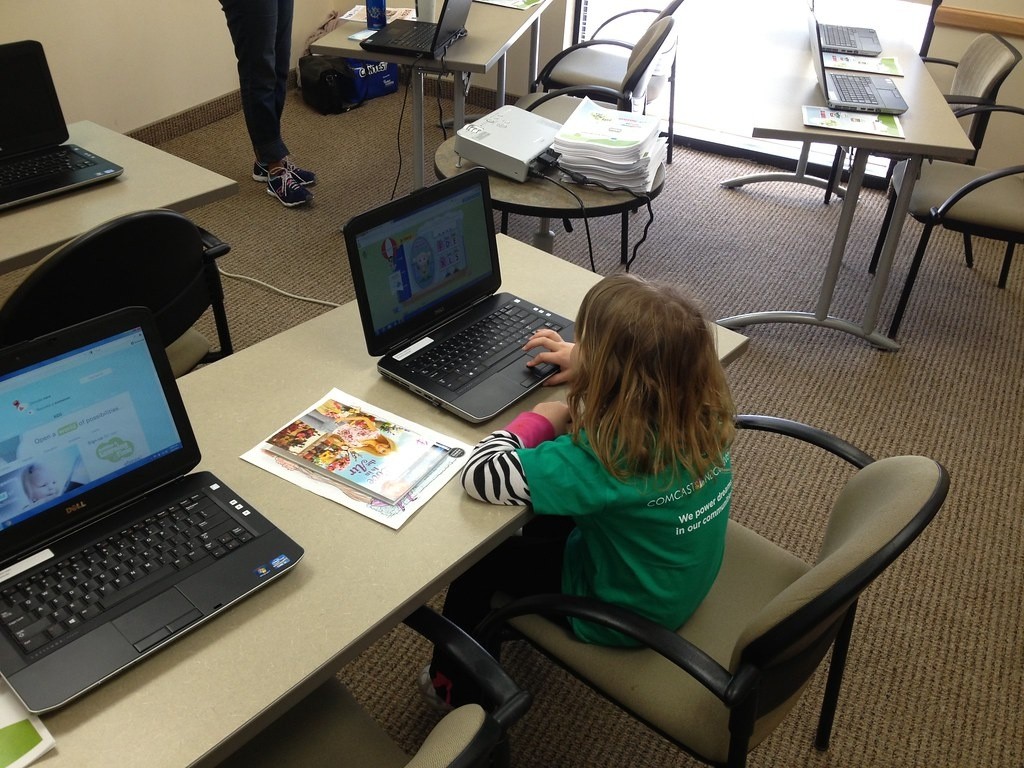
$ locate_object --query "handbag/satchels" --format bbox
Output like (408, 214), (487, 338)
(299, 55), (361, 115)
(345, 58), (399, 100)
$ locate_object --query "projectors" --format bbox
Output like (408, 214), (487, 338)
(454, 105), (563, 182)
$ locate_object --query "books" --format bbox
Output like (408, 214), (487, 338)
(822, 52), (904, 77)
(266, 399), (451, 505)
(554, 96), (670, 193)
(472, 0), (543, 10)
(802, 105), (905, 138)
(0, 674), (56, 768)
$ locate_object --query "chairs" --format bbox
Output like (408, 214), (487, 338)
(866, 103), (1024, 356)
(513, 17), (675, 215)
(824, 31), (1024, 270)
(530, 0), (684, 164)
(215, 603), (531, 768)
(471, 415), (950, 768)
(0, 209), (236, 379)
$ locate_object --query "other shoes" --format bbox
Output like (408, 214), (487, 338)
(419, 665), (455, 713)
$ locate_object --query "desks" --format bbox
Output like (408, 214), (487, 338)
(0, 120), (238, 279)
(433, 131), (665, 266)
(0, 228), (752, 768)
(309, 0), (553, 196)
(715, 22), (975, 352)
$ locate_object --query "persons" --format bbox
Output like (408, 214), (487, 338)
(418, 274), (738, 715)
(21, 461), (62, 502)
(219, 0), (317, 207)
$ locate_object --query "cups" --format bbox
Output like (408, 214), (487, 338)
(366, 0), (386, 31)
(414, 0), (435, 22)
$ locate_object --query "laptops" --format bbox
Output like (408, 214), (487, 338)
(806, 0), (928, 109)
(0, 40), (125, 211)
(0, 305), (305, 713)
(359, 0), (473, 59)
(343, 166), (580, 421)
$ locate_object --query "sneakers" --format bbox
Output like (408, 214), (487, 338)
(266, 168), (313, 206)
(253, 160), (317, 185)
